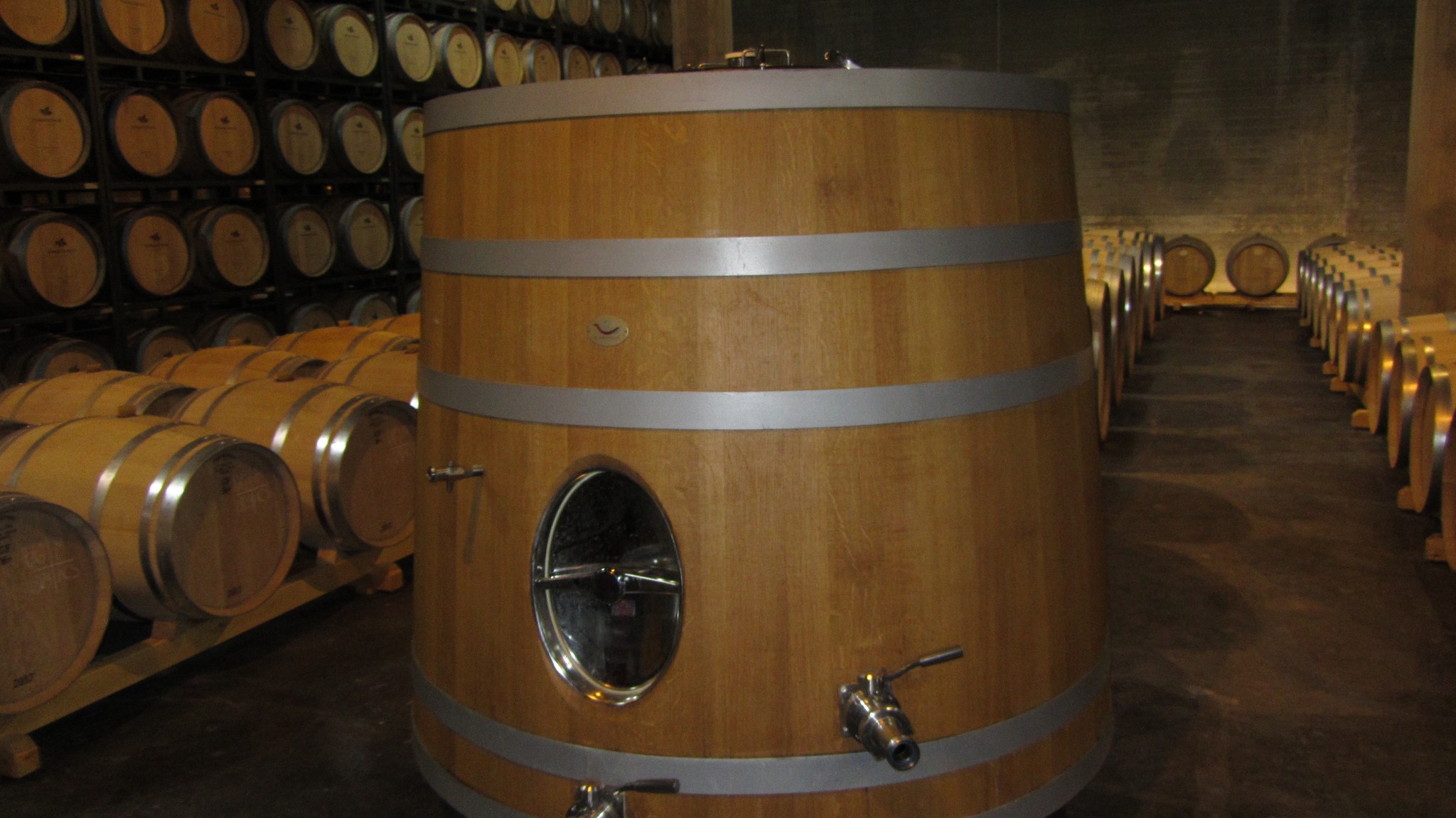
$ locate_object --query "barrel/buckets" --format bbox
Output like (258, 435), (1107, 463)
(405, 69), (1116, 817)
(1081, 229), (1456, 571)
(0, 1), (677, 721)
